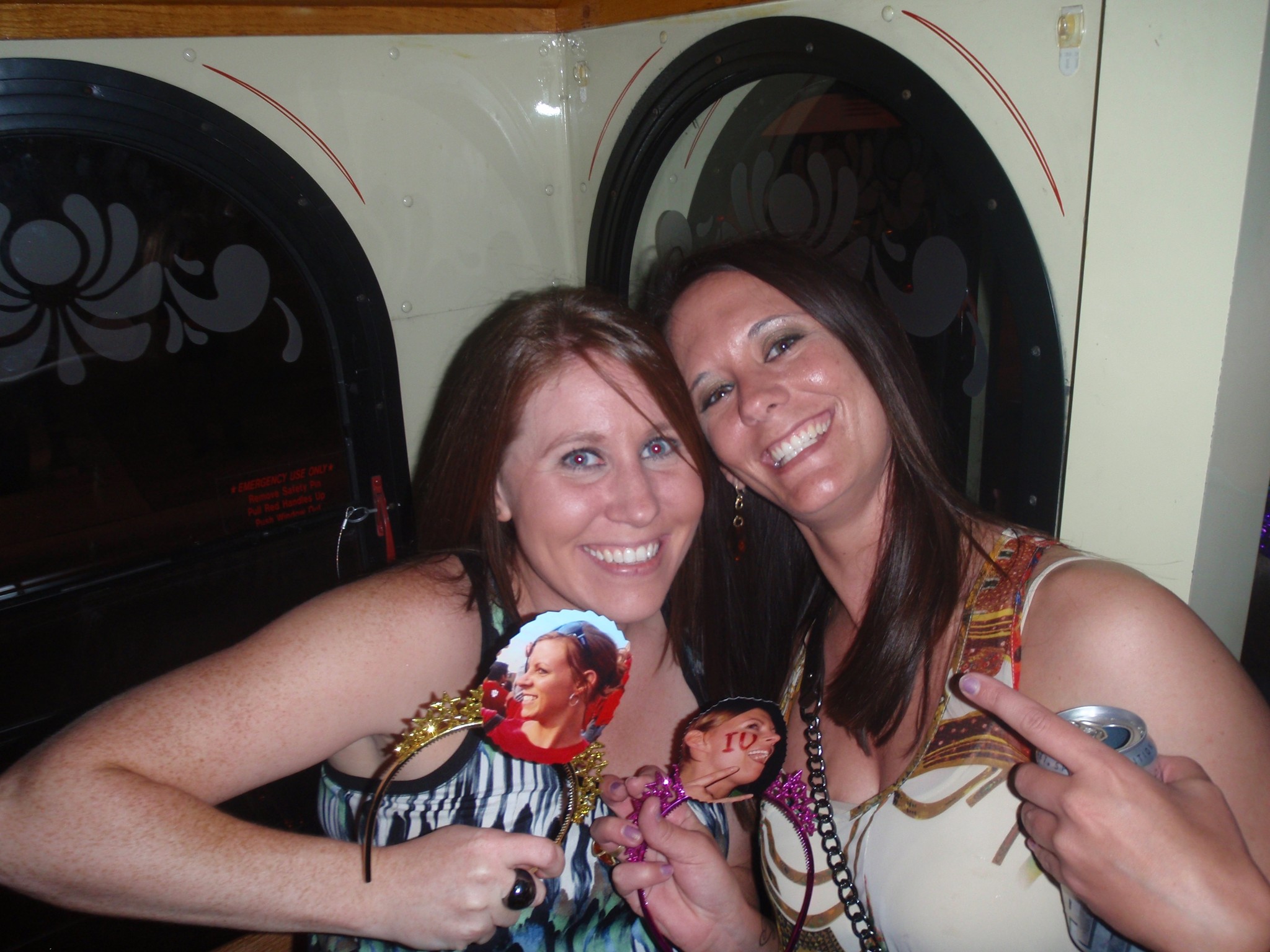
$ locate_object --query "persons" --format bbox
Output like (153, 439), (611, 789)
(582, 647), (632, 743)
(677, 705), (781, 803)
(590, 239), (1270, 952)
(480, 661), (512, 715)
(481, 621), (620, 763)
(0, 286), (759, 952)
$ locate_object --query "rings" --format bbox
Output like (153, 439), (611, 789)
(501, 869), (537, 910)
(611, 844), (625, 858)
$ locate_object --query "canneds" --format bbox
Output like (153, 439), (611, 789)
(1035, 704), (1165, 952)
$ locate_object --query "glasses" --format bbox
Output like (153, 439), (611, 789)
(553, 621), (599, 674)
